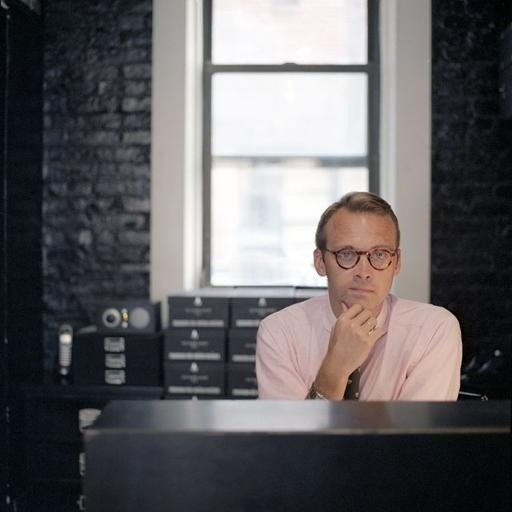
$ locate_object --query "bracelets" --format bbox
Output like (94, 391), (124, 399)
(310, 386), (327, 399)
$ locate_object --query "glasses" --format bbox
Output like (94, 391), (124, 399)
(317, 244), (399, 270)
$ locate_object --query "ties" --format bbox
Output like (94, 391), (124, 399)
(345, 368), (361, 400)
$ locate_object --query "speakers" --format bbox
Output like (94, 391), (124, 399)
(58, 324), (74, 375)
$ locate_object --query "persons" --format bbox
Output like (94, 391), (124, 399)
(256, 193), (463, 402)
